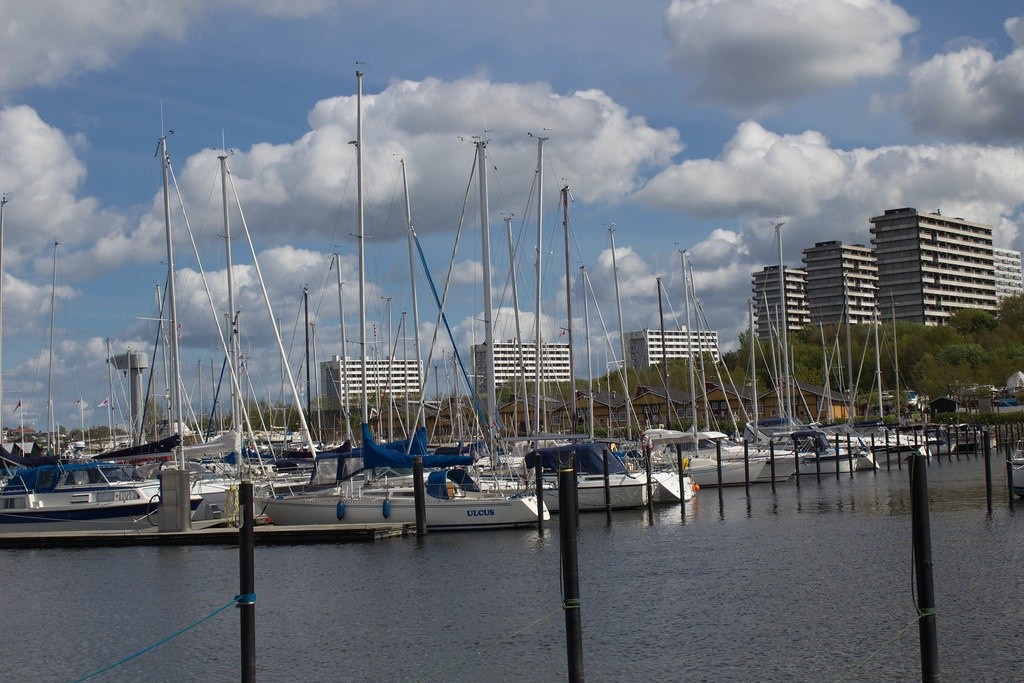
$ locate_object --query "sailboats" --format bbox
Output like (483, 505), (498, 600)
(0, 67), (983, 528)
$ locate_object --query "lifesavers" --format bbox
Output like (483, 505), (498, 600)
(682, 458), (689, 471)
(610, 443), (619, 453)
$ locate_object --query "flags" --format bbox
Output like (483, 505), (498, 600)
(176, 320), (182, 338)
